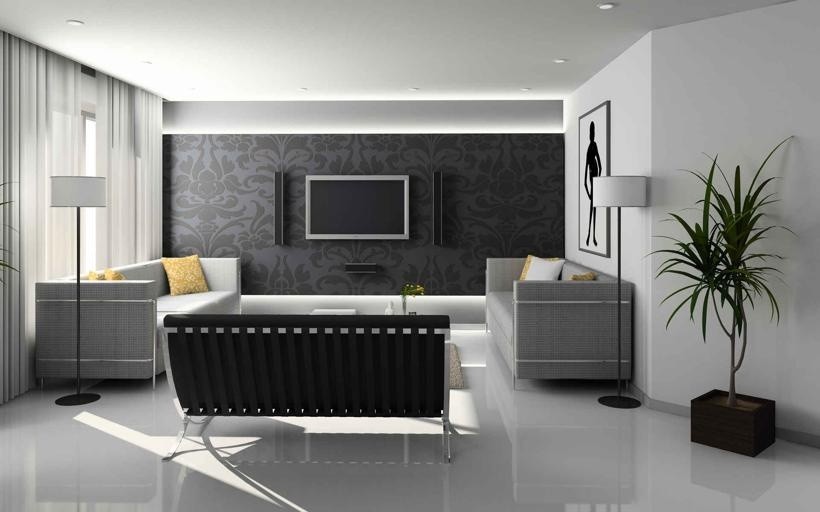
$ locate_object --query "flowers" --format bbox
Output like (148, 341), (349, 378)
(401, 283), (425, 299)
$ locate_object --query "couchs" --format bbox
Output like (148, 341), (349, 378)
(34, 257), (242, 389)
(485, 256), (633, 392)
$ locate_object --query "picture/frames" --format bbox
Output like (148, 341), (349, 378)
(577, 100), (612, 258)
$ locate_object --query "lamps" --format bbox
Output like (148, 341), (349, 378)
(592, 175), (648, 410)
(48, 175), (107, 406)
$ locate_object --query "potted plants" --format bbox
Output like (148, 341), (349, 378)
(638, 132), (797, 459)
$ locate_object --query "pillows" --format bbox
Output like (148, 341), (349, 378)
(567, 271), (600, 281)
(87, 267), (127, 280)
(518, 254), (566, 281)
(161, 254), (209, 296)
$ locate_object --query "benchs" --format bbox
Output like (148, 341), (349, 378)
(160, 314), (452, 463)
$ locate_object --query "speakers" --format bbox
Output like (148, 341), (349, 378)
(274, 171), (283, 246)
(343, 263), (377, 274)
(433, 172), (442, 247)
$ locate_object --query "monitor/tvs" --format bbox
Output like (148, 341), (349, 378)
(305, 174), (410, 240)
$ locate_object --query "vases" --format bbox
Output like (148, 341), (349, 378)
(402, 297), (407, 315)
